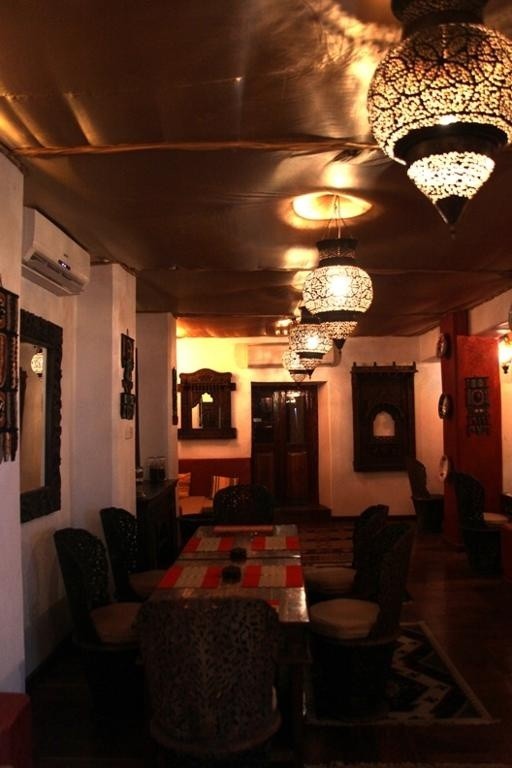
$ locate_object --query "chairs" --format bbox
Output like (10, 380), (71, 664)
(99, 507), (164, 599)
(213, 481), (272, 526)
(308, 520), (415, 725)
(180, 476), (240, 517)
(453, 471), (508, 577)
(135, 595), (287, 768)
(404, 454), (444, 533)
(303, 504), (389, 595)
(177, 472), (192, 500)
(53, 528), (140, 706)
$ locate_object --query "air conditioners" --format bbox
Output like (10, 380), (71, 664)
(23, 205), (94, 298)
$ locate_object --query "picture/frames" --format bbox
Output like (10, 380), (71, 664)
(436, 332), (450, 358)
(439, 393), (452, 418)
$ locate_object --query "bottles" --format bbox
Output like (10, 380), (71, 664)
(148, 457), (166, 490)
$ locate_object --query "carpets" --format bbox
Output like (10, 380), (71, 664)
(300, 620), (502, 726)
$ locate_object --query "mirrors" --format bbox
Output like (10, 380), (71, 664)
(20, 308), (64, 523)
(177, 368), (237, 440)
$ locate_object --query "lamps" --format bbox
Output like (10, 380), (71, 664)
(280, 346), (308, 387)
(499, 335), (511, 373)
(290, 322), (334, 378)
(365, 0), (512, 226)
(302, 194), (373, 349)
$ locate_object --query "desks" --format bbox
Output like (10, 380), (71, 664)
(177, 524), (302, 559)
(150, 558), (311, 768)
